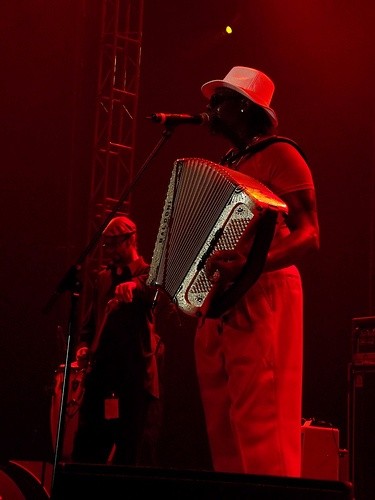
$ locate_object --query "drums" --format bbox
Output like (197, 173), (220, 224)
(52, 364), (118, 463)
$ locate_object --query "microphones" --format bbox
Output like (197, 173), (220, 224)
(144, 111), (209, 126)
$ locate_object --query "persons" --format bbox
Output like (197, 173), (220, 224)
(194, 66), (321, 478)
(72, 216), (160, 465)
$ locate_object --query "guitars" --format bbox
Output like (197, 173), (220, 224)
(75, 297), (120, 395)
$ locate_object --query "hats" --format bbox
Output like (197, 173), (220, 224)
(101, 216), (137, 238)
(200, 65), (279, 129)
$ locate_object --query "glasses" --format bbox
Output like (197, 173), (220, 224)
(209, 95), (243, 106)
(98, 235), (130, 249)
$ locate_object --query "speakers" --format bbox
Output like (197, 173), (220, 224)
(53, 360), (375, 500)
